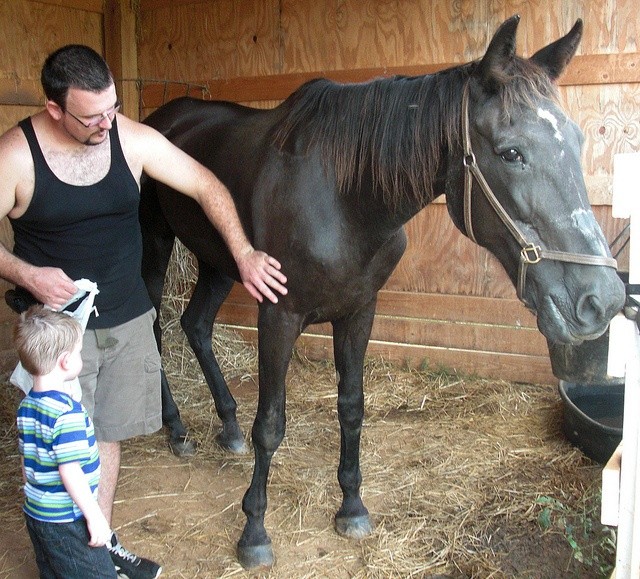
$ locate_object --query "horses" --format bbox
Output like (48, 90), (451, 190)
(138, 14), (626, 572)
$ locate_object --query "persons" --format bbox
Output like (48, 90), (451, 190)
(13, 303), (118, 579)
(0, 44), (288, 579)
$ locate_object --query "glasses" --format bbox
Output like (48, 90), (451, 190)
(58, 103), (122, 129)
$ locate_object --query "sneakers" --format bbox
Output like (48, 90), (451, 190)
(106, 533), (161, 579)
